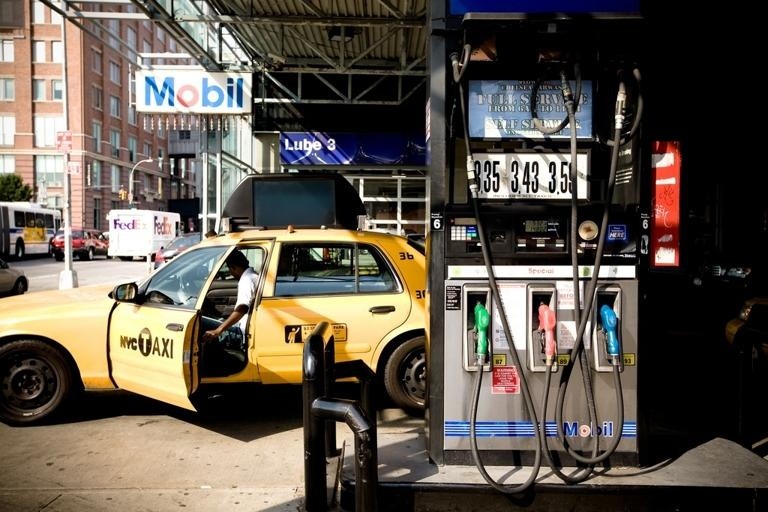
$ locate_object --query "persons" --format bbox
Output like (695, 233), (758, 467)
(201, 251), (259, 350)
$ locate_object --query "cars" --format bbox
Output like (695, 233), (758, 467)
(0, 259), (28, 296)
(0, 219), (432, 427)
(155, 231), (203, 269)
(50, 227), (108, 260)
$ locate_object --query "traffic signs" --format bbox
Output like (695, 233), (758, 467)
(57, 131), (73, 154)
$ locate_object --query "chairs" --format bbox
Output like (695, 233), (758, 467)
(377, 267), (391, 281)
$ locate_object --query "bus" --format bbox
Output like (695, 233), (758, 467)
(0, 200), (63, 262)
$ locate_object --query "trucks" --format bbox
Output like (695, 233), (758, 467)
(106, 209), (181, 262)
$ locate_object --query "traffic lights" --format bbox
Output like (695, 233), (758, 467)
(118, 189), (127, 201)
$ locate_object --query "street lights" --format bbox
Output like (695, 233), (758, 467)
(128, 159), (154, 209)
